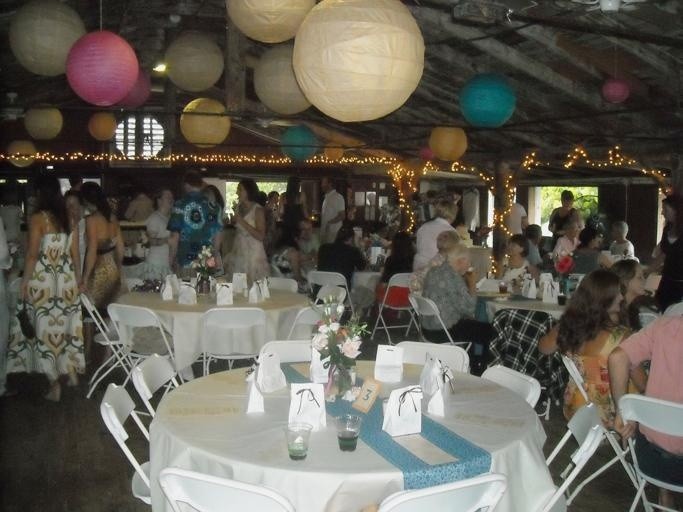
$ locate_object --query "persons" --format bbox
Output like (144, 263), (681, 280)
(537, 270), (648, 447)
(409, 230), (459, 298)
(375, 231), (417, 307)
(6, 176), (86, 403)
(260, 191), (288, 218)
(497, 234), (530, 295)
(522, 224), (553, 270)
(141, 186), (176, 282)
(547, 189), (583, 253)
(607, 314), (683, 512)
(227, 178), (271, 285)
(122, 182), (154, 222)
(266, 233), (310, 293)
(319, 175), (345, 245)
(61, 190), (85, 222)
(313, 226), (365, 328)
(1, 213), (21, 400)
(80, 182), (124, 367)
(571, 228), (614, 275)
(607, 259), (645, 332)
(607, 220), (634, 259)
(551, 220), (578, 260)
(165, 171), (225, 281)
(640, 194), (683, 315)
(412, 200), (461, 273)
(504, 189), (529, 239)
(419, 244), (498, 377)
(277, 176), (309, 240)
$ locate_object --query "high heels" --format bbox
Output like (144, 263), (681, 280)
(43, 386), (62, 402)
(66, 374), (79, 386)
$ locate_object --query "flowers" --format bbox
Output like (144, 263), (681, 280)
(306, 294), (371, 399)
(549, 246), (578, 275)
(188, 243), (217, 291)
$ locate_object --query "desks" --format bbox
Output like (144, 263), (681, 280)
(132, 360), (569, 512)
(476, 288), (576, 323)
(118, 290), (314, 381)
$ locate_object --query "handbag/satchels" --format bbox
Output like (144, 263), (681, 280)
(15, 299), (34, 339)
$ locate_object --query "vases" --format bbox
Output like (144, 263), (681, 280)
(197, 276), (210, 293)
(325, 362), (354, 396)
(555, 273), (566, 291)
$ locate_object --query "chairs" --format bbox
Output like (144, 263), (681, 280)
(80, 293), (131, 400)
(478, 364), (541, 410)
(378, 471), (512, 512)
(261, 340), (313, 366)
(131, 353), (179, 417)
(315, 284), (345, 305)
(306, 271), (356, 321)
(408, 295), (473, 355)
(487, 310), (569, 420)
(101, 383), (153, 505)
(108, 304), (185, 419)
(263, 277), (297, 291)
(561, 357), (642, 498)
(395, 341), (469, 375)
(285, 304), (344, 338)
(618, 393), (683, 512)
(371, 273), (422, 344)
(159, 469), (296, 512)
(200, 308), (274, 377)
(511, 401), (605, 512)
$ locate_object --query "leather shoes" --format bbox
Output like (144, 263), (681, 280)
(2, 385), (18, 397)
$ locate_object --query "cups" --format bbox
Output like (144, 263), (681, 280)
(283, 422), (313, 462)
(465, 267), (474, 276)
(335, 415), (361, 453)
(557, 289), (565, 305)
(498, 281), (507, 294)
